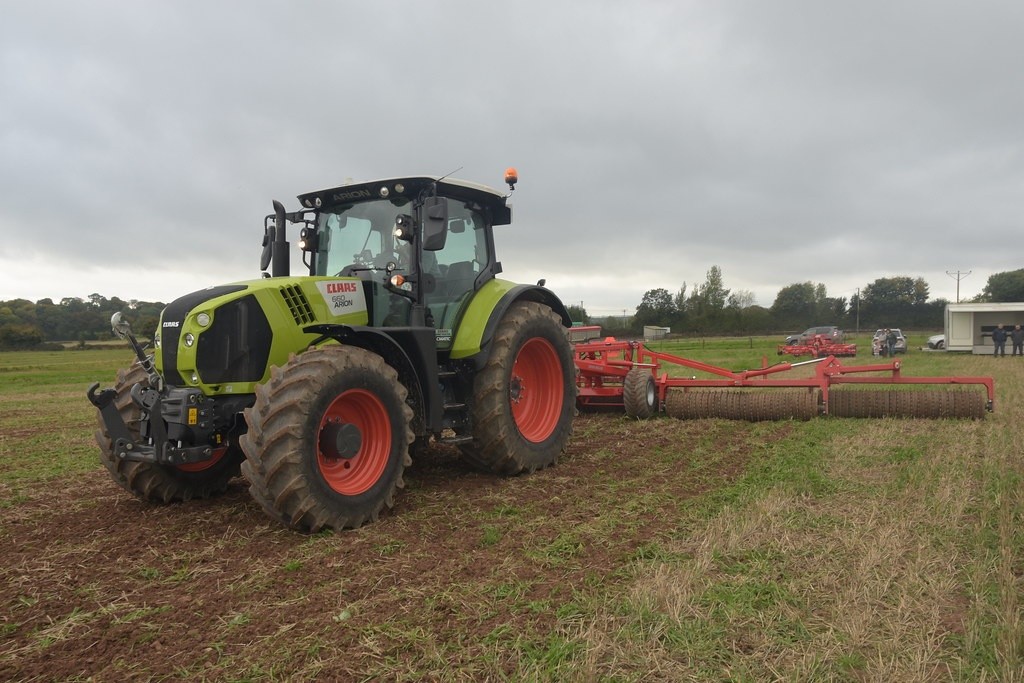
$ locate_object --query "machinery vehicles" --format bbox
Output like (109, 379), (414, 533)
(87, 165), (995, 537)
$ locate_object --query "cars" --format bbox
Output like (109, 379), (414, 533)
(785, 326), (843, 347)
(870, 327), (908, 356)
(927, 333), (946, 349)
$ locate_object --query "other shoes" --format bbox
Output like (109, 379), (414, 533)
(994, 355), (997, 357)
(1011, 354), (1016, 357)
(1020, 354), (1023, 356)
(1002, 355), (1004, 357)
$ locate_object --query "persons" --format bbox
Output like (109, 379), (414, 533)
(991, 322), (1008, 358)
(394, 240), (444, 279)
(1009, 322), (1024, 357)
(877, 327), (899, 360)
